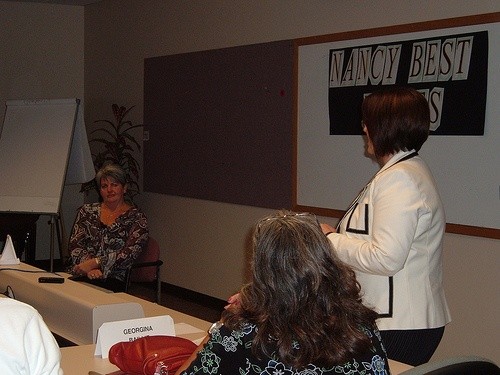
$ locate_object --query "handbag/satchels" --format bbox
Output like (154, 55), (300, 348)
(109, 336), (199, 375)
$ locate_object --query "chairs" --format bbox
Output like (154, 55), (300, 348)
(126, 238), (166, 304)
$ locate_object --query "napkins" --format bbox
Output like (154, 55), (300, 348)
(0, 234), (20, 265)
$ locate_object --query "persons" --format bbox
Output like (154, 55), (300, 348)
(173, 211), (391, 375)
(0, 294), (63, 375)
(64, 165), (148, 292)
(318, 84), (451, 367)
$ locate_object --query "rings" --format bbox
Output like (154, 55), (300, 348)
(91, 274), (95, 276)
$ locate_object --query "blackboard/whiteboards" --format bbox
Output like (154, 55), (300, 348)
(0, 98), (80, 216)
(295, 12), (499, 239)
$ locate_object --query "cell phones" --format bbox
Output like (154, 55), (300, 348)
(39, 277), (64, 283)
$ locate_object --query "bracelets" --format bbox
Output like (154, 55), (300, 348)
(95, 258), (99, 264)
(208, 322), (223, 335)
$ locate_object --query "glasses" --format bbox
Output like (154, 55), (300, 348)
(256, 211), (321, 233)
(361, 120), (367, 128)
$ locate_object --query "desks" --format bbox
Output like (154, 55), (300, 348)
(0, 257), (213, 375)
